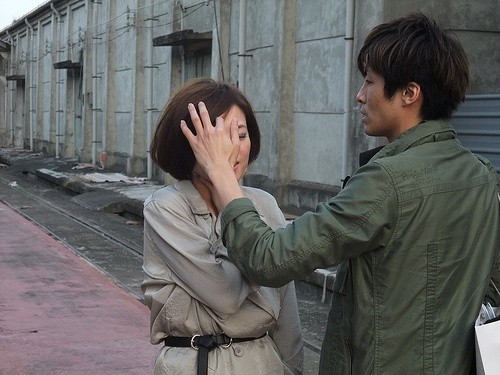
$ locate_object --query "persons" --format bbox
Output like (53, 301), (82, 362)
(135, 77), (305, 374)
(178, 11), (500, 375)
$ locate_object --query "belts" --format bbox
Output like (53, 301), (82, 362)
(164, 333), (268, 375)
